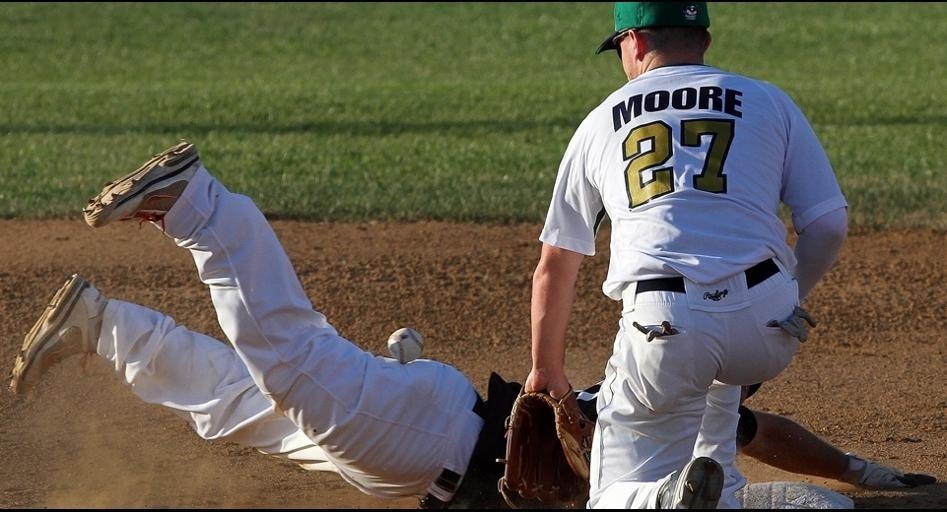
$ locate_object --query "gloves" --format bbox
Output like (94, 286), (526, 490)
(632, 321), (678, 343)
(767, 306), (820, 343)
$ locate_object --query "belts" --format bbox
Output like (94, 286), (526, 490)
(631, 257), (778, 293)
(416, 389), (489, 511)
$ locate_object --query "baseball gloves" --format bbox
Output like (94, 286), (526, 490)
(495, 384), (594, 510)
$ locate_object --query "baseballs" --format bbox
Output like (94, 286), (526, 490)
(387, 327), (424, 364)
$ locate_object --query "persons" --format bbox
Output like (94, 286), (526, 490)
(495, 2), (850, 509)
(5, 138), (936, 509)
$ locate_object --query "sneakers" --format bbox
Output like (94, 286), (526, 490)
(83, 139), (203, 232)
(860, 459), (939, 492)
(655, 455), (725, 511)
(10, 272), (109, 398)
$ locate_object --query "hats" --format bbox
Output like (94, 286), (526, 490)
(593, 3), (711, 57)
